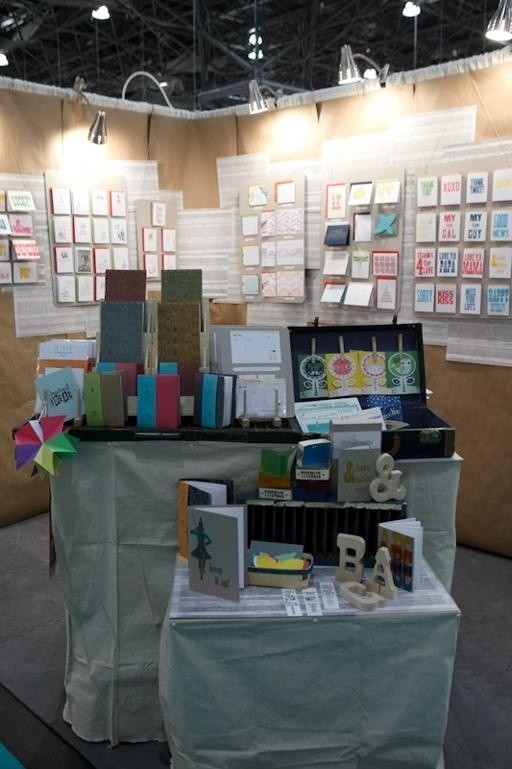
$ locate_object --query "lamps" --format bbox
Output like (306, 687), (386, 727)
(481, 0), (512, 47)
(338, 43), (390, 85)
(72, 76), (109, 149)
(246, 78), (284, 117)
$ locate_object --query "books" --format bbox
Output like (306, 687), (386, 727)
(33, 269), (237, 430)
(176, 475), (233, 559)
(377, 515), (424, 594)
(255, 393), (405, 505)
(186, 504), (248, 604)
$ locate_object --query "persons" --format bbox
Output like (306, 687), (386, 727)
(189, 521), (213, 580)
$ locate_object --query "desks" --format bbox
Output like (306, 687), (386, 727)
(39, 406), (463, 751)
(155, 550), (462, 769)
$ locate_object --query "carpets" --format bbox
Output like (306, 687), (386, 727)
(0, 511), (511, 769)
